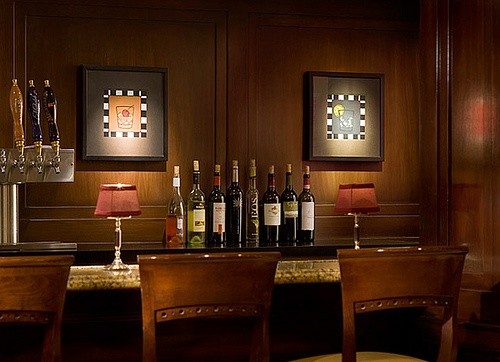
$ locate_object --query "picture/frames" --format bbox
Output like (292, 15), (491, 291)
(306, 70), (385, 162)
(80, 63), (169, 162)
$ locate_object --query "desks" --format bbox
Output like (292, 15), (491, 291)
(65, 254), (342, 291)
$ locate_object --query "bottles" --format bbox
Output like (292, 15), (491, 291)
(165, 166), (187, 248)
(281, 164), (298, 244)
(226, 160), (244, 245)
(261, 164), (282, 243)
(298, 165), (316, 242)
(245, 159), (261, 243)
(207, 165), (227, 243)
(187, 160), (207, 248)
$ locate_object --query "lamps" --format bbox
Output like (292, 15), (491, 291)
(93, 183), (142, 271)
(334, 182), (381, 249)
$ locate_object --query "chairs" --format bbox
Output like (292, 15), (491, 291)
(284, 242), (469, 362)
(138, 250), (282, 362)
(0, 254), (75, 362)
